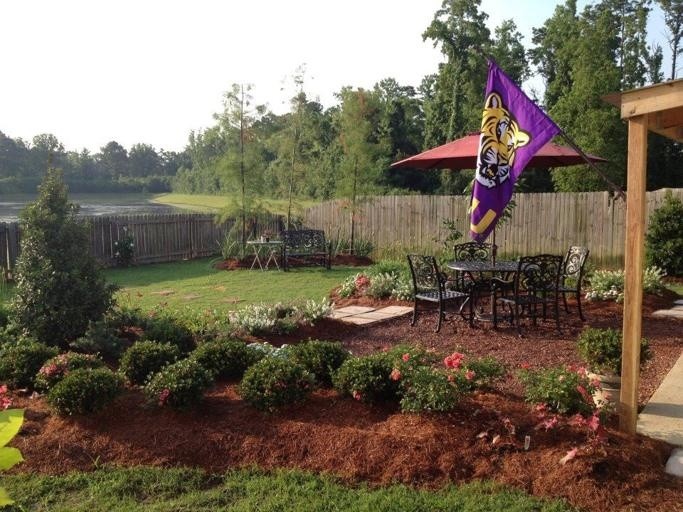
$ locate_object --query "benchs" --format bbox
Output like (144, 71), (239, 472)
(279, 227), (335, 272)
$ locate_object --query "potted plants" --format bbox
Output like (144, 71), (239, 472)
(579, 321), (652, 415)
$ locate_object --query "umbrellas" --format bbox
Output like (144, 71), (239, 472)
(388, 130), (612, 266)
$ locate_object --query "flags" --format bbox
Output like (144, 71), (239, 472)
(459, 60), (564, 246)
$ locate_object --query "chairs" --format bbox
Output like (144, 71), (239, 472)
(406, 241), (591, 337)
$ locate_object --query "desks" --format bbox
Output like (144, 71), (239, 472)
(244, 239), (283, 271)
